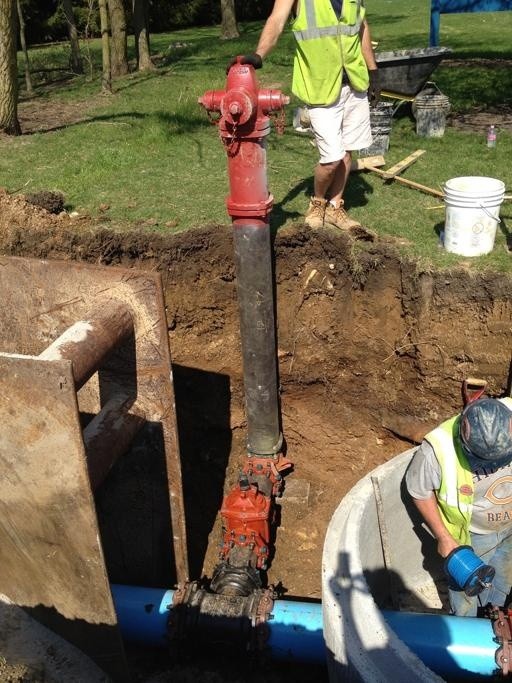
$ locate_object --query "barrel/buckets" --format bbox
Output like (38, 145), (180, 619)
(442, 176), (505, 256)
(358, 101), (393, 156)
(412, 81), (451, 139)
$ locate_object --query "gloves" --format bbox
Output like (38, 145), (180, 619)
(226, 53), (262, 76)
(368, 69), (381, 108)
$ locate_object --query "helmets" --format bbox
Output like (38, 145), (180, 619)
(458, 398), (512, 460)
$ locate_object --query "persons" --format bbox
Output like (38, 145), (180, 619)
(255, 0), (381, 231)
(405, 396), (512, 617)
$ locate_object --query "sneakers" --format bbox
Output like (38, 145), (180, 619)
(304, 195), (329, 234)
(324, 199), (362, 231)
